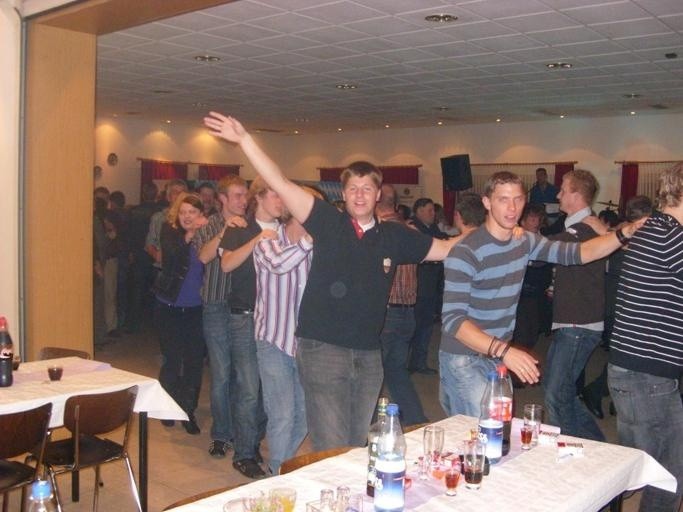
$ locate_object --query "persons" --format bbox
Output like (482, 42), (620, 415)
(92, 110), (682, 512)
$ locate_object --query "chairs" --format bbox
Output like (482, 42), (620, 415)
(21, 385), (145, 512)
(0, 402), (53, 512)
(401, 422), (429, 433)
(164, 480), (248, 510)
(39, 346), (91, 363)
(279, 446), (352, 475)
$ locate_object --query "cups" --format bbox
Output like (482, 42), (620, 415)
(270, 488), (296, 512)
(464, 440), (485, 489)
(319, 485), (364, 511)
(12, 355), (20, 371)
(47, 363), (63, 381)
(524, 404), (541, 445)
(424, 427), (444, 466)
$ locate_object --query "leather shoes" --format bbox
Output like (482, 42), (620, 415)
(232, 458), (266, 481)
(410, 365), (438, 376)
(208, 439), (227, 460)
(257, 450), (264, 465)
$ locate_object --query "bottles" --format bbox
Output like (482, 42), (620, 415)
(488, 365), (513, 456)
(29, 481), (55, 512)
(0, 318), (13, 387)
(367, 397), (389, 497)
(374, 404), (406, 512)
(478, 371), (502, 464)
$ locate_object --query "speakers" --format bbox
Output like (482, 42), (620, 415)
(440, 154), (472, 191)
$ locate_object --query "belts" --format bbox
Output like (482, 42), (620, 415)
(385, 302), (417, 309)
(230, 308), (255, 316)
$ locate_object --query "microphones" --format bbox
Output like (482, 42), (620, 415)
(535, 181), (539, 187)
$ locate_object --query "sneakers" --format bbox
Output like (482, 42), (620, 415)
(181, 415), (201, 435)
(159, 420), (175, 429)
(582, 383), (604, 420)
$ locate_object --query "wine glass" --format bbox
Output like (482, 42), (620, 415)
(445, 459), (460, 495)
(521, 426), (532, 449)
